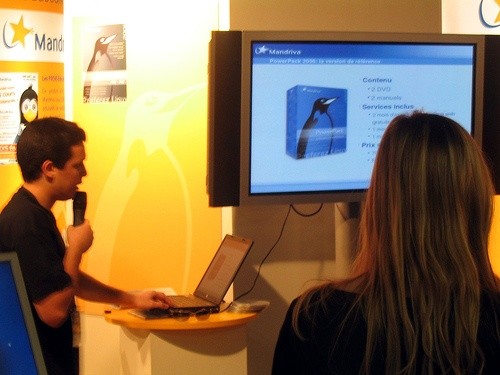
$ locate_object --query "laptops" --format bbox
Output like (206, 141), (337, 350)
(150, 234), (253, 314)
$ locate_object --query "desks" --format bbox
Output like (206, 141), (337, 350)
(106, 309), (259, 375)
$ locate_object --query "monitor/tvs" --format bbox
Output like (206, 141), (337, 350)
(206, 31), (500, 208)
(0, 252), (48, 375)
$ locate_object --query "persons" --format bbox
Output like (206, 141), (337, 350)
(272, 113), (500, 375)
(0, 114), (171, 375)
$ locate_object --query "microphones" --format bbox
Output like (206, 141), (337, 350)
(73, 192), (87, 226)
